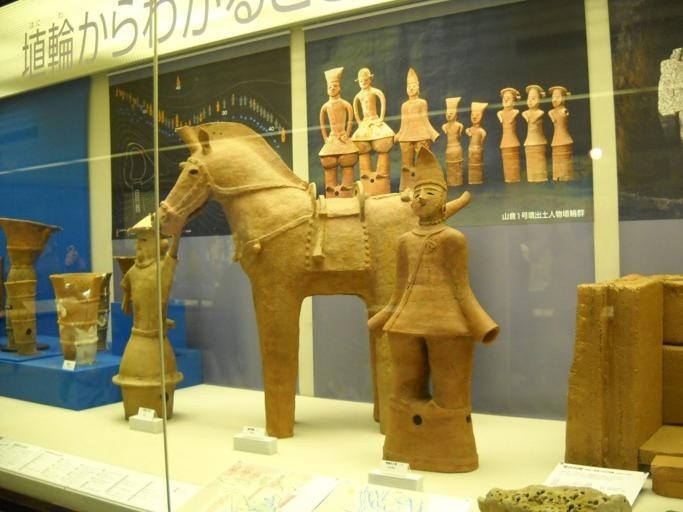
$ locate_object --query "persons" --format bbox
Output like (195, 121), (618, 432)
(318, 66), (574, 198)
(119, 228), (181, 380)
(367, 146), (499, 409)
